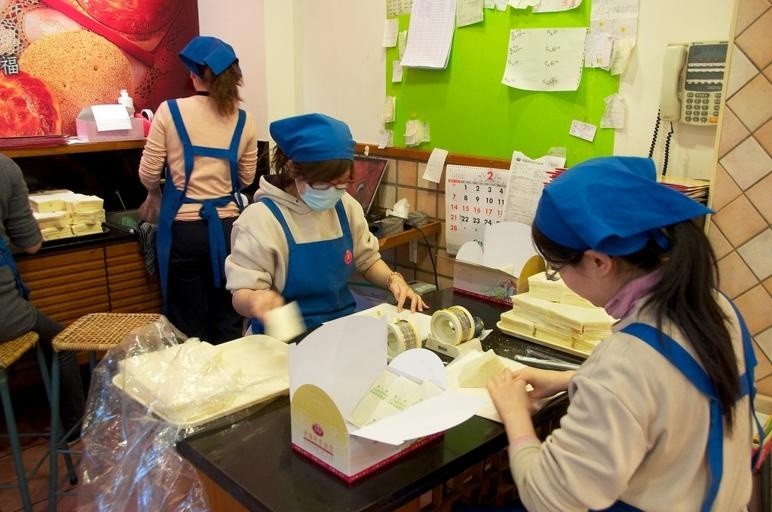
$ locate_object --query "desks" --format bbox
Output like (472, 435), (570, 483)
(175, 286), (586, 512)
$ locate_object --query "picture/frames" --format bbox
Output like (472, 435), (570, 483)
(353, 156), (389, 215)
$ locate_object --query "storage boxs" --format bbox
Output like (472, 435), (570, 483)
(76, 118), (144, 142)
(289, 315), (484, 484)
(453, 221), (545, 306)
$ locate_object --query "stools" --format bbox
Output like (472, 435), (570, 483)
(0, 332), (78, 512)
(49, 312), (163, 512)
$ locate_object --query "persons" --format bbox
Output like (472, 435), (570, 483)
(225, 112), (430, 338)
(486, 157), (765, 512)
(139, 35), (258, 345)
(0, 154), (89, 442)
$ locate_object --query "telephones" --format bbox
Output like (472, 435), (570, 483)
(657, 40), (732, 127)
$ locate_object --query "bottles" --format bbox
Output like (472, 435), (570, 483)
(116, 88), (137, 121)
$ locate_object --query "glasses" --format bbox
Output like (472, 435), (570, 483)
(544, 256), (580, 280)
(300, 171), (353, 191)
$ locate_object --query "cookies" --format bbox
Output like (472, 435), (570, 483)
(17, 32), (133, 135)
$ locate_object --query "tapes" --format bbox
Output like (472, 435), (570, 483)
(387, 319), (418, 357)
(431, 305), (476, 345)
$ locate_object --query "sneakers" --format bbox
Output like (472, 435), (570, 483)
(60, 429), (81, 447)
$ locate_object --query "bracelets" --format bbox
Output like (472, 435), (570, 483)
(384, 272), (403, 290)
